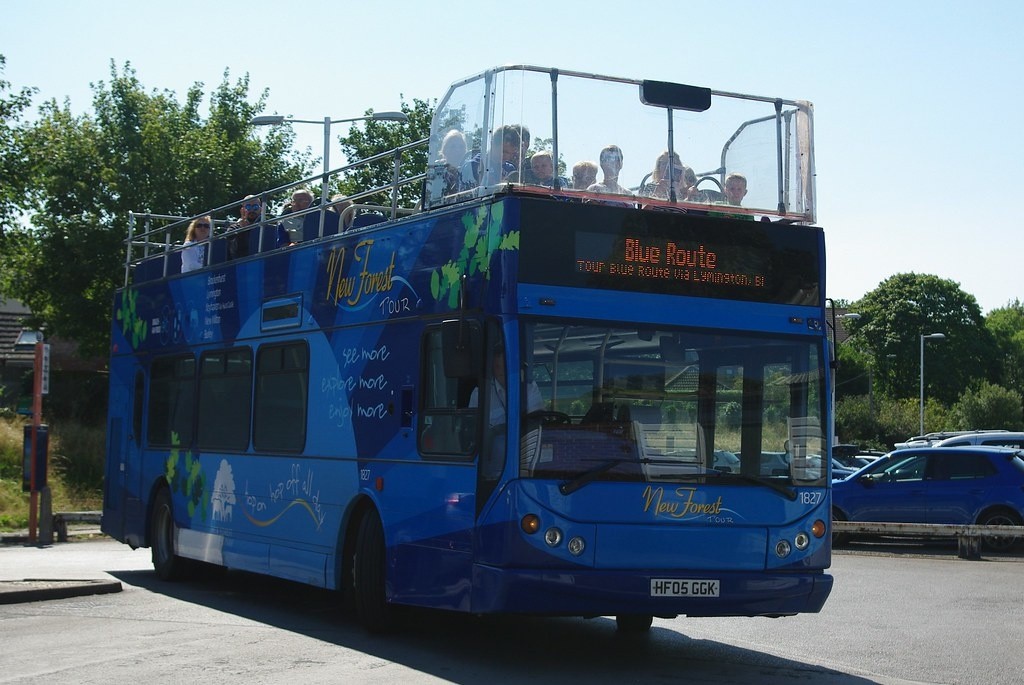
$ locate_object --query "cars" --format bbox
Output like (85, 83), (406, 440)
(666, 429), (1024, 553)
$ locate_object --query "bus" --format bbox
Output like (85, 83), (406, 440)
(100, 65), (837, 635)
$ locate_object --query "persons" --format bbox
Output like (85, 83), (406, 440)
(468, 343), (543, 429)
(181, 216), (211, 274)
(435, 124), (748, 207)
(226, 194), (261, 260)
(281, 190), (355, 247)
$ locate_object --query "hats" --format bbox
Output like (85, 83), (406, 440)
(658, 151), (686, 172)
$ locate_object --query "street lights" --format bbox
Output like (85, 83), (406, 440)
(920, 333), (946, 435)
(832, 313), (861, 446)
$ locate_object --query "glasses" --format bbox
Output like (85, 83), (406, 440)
(194, 223), (210, 229)
(684, 175), (695, 183)
(520, 142), (529, 148)
(243, 204), (262, 211)
